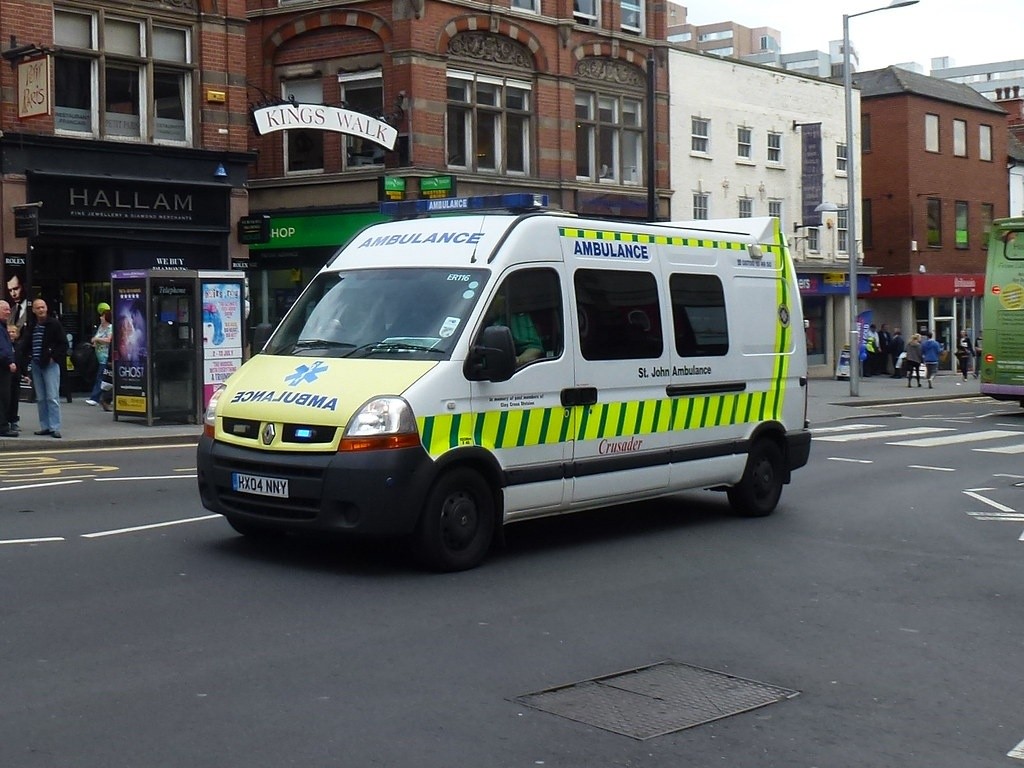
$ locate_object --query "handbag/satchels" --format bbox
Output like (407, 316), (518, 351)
(895, 352), (911, 371)
(939, 349), (950, 363)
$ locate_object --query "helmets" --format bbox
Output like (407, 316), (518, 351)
(96, 302), (110, 315)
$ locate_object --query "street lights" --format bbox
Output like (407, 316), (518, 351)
(813, 0), (919, 397)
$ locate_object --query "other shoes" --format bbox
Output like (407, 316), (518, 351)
(964, 379), (967, 381)
(101, 400), (113, 412)
(973, 374), (977, 379)
(908, 386), (911, 388)
(86, 400), (99, 407)
(34, 428), (50, 435)
(0, 424), (22, 437)
(50, 431), (62, 438)
(929, 387), (933, 389)
(917, 384), (921, 387)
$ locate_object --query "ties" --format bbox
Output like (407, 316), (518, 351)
(13, 304), (22, 326)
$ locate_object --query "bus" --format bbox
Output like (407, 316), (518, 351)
(979, 216), (1024, 409)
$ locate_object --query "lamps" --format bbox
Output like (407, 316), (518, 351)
(722, 176), (730, 189)
(792, 217), (835, 231)
(759, 180), (765, 193)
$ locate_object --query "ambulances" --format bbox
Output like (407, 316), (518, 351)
(197, 193), (811, 575)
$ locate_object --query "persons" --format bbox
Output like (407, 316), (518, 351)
(20, 297), (70, 438)
(85, 310), (113, 407)
(903, 333), (924, 389)
(462, 283), (546, 370)
(5, 269), (32, 339)
(115, 299), (147, 363)
(862, 324), (905, 380)
(956, 329), (976, 383)
(921, 331), (944, 388)
(974, 330), (985, 379)
(0, 299), (21, 436)
